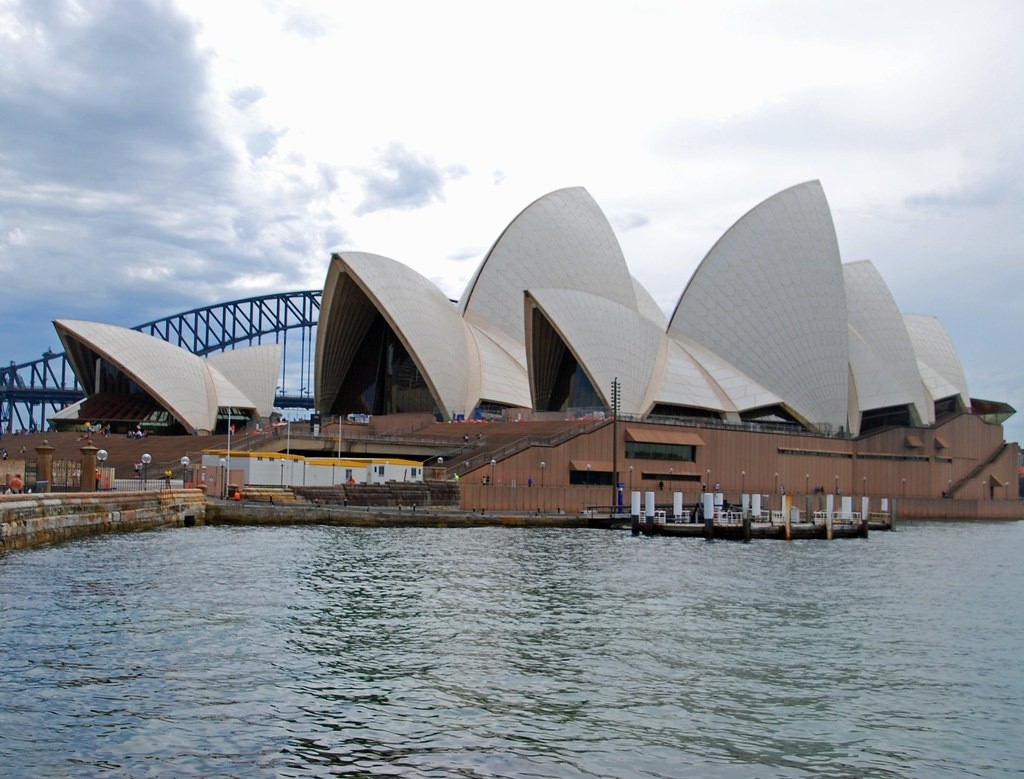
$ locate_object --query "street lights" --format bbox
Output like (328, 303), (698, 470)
(97, 450), (108, 489)
(180, 457), (190, 488)
(141, 453), (152, 490)
(218, 458), (226, 501)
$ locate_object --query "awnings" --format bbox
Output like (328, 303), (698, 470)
(570, 460), (622, 472)
(625, 428), (706, 446)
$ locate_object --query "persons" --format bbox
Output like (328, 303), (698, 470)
(0, 448), (8, 460)
(96, 469), (101, 491)
(814, 485), (825, 495)
(74, 421), (110, 441)
(165, 468), (172, 489)
(715, 482), (720, 491)
(9, 474), (23, 494)
(234, 490), (240, 506)
(230, 424), (235, 435)
(481, 474), (490, 485)
(135, 460), (143, 479)
(3, 423), (58, 434)
(128, 427), (148, 440)
(348, 476), (355, 484)
(703, 483), (706, 491)
(528, 476), (532, 487)
(659, 480), (663, 490)
(21, 445), (26, 453)
(454, 473), (460, 482)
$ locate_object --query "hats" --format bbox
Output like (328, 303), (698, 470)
(9, 478), (22, 489)
(15, 473), (21, 477)
(95, 468), (98, 471)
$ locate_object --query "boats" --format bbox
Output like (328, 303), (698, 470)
(638, 520), (863, 539)
